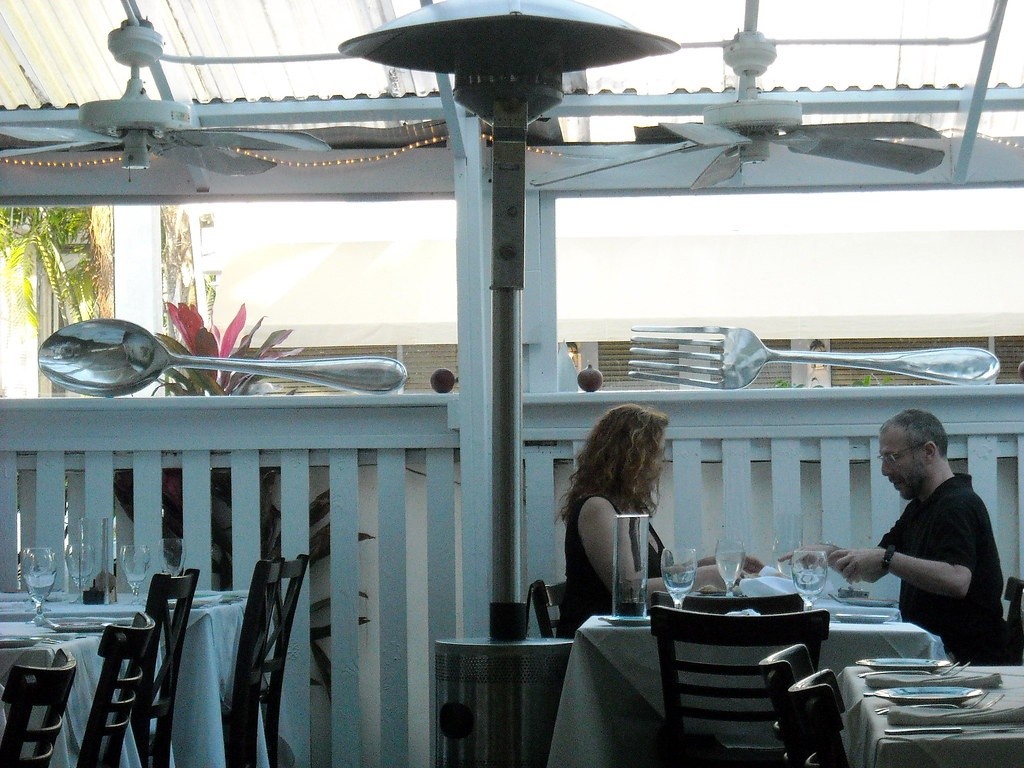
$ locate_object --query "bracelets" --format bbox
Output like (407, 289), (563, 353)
(881, 545), (894, 572)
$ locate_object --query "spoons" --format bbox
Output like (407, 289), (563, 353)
(34, 318), (406, 393)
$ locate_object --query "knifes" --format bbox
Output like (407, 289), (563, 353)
(884, 727), (1015, 735)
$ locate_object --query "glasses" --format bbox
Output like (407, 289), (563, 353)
(876, 439), (938, 465)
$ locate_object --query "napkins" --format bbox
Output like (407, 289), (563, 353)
(864, 673), (1001, 689)
(887, 707), (1024, 726)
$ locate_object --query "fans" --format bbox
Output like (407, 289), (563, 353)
(530, 0), (946, 199)
(0, 1), (332, 175)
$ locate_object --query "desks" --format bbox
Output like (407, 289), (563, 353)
(546, 563), (935, 768)
(0, 622), (140, 768)
(835, 667), (1024, 768)
(0, 590), (267, 768)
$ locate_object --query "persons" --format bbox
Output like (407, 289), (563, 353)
(778, 410), (1007, 664)
(555, 406), (763, 638)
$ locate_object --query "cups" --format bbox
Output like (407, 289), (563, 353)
(80, 517), (112, 606)
(611, 514), (649, 617)
(157, 538), (187, 579)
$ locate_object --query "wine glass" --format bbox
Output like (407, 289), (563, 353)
(791, 550), (827, 612)
(20, 549), (57, 628)
(120, 543), (150, 608)
(713, 536), (745, 598)
(64, 541), (94, 604)
(660, 547), (696, 608)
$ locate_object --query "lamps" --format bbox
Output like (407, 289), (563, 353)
(731, 128), (769, 165)
(118, 130), (160, 171)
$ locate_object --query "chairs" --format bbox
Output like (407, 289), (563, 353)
(77, 610), (158, 768)
(530, 580), (573, 637)
(129, 567), (203, 768)
(0, 648), (77, 767)
(255, 553), (312, 767)
(998, 577), (1024, 663)
(648, 590), (848, 767)
(221, 555), (286, 766)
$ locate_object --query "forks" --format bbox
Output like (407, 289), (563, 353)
(629, 324), (1000, 385)
(854, 655), (1022, 735)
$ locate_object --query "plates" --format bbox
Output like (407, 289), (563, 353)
(823, 598), (900, 625)
(598, 616), (651, 626)
(856, 657), (953, 674)
(875, 686), (983, 707)
(0, 595), (248, 649)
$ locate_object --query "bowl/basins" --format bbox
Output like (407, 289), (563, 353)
(837, 588), (868, 600)
